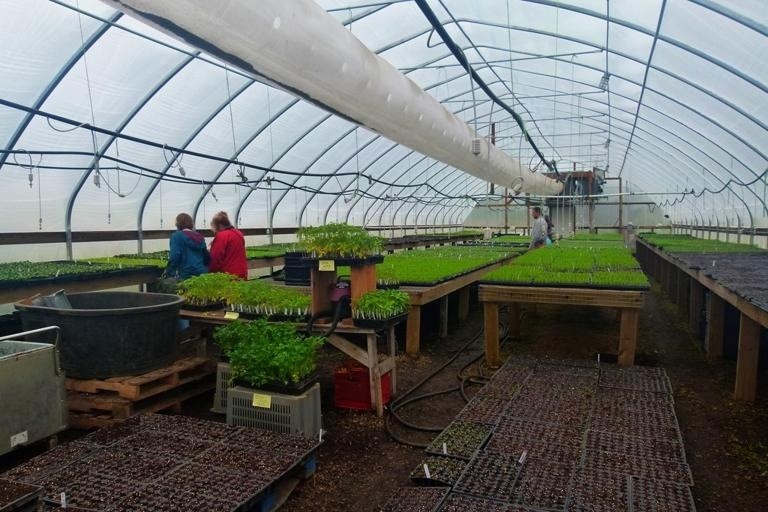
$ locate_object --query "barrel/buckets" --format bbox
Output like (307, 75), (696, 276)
(283, 250), (317, 286)
(484, 228), (492, 239)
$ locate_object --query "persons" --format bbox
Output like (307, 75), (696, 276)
(207, 210), (249, 280)
(161, 213), (211, 283)
(528, 206), (548, 249)
(544, 216), (554, 241)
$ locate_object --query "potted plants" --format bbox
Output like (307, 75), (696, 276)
(212, 318), (326, 397)
(349, 286), (412, 330)
(296, 222), (385, 270)
(177, 271), (311, 321)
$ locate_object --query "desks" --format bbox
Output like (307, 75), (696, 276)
(405, 237), (650, 369)
(179, 309), (401, 418)
(636, 237), (768, 404)
